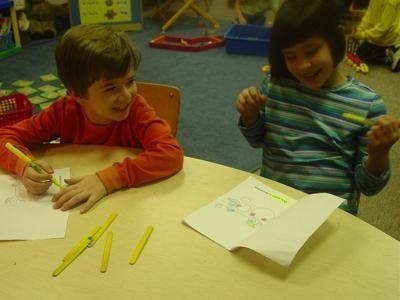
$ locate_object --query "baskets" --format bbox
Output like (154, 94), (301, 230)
(0, 91), (35, 127)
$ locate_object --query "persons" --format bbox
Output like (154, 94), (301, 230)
(235, 0), (282, 29)
(1, 23), (183, 214)
(234, 1), (400, 221)
(353, 0), (400, 72)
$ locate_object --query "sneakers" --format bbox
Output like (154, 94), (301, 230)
(389, 45), (400, 71)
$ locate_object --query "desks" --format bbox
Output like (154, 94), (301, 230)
(0, 145), (400, 300)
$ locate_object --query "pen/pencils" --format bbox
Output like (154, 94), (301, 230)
(342, 113), (376, 127)
(5, 142), (66, 188)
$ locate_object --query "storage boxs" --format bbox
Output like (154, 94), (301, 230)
(224, 24), (274, 56)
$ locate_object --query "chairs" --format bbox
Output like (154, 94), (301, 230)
(136, 82), (180, 138)
